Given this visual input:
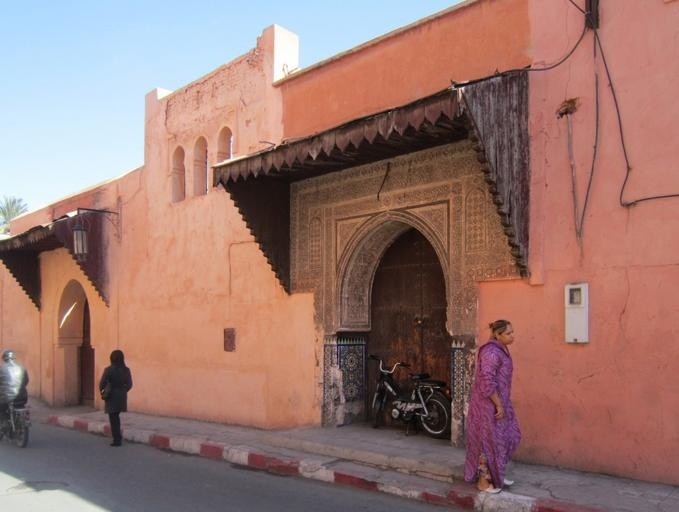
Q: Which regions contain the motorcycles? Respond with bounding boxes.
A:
[0,400,30,447]
[367,354,451,439]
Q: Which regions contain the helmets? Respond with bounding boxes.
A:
[2,350,17,360]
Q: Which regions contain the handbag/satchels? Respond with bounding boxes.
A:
[100,382,114,400]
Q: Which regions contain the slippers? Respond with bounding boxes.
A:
[476,482,502,494]
[501,478,515,486]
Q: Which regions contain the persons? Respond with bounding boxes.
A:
[0,350,29,435]
[99,350,133,447]
[461,319,524,494]
